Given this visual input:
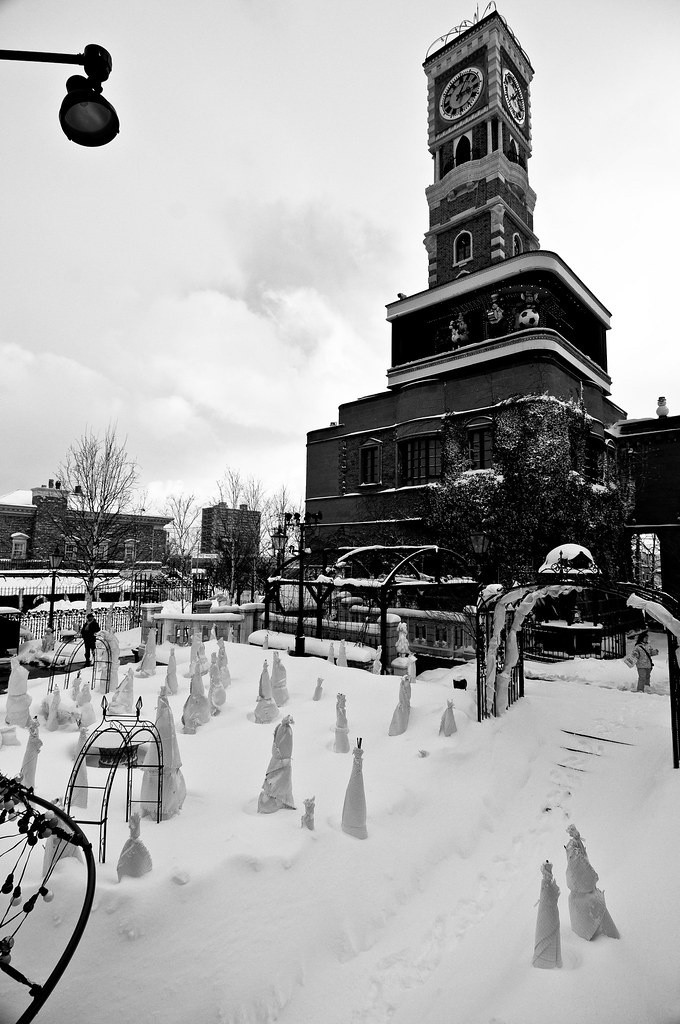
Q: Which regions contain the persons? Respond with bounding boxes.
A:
[81,612,100,662]
[632,632,659,693]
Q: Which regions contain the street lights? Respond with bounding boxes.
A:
[0,43,121,148]
[47,544,65,630]
[270,524,289,615]
